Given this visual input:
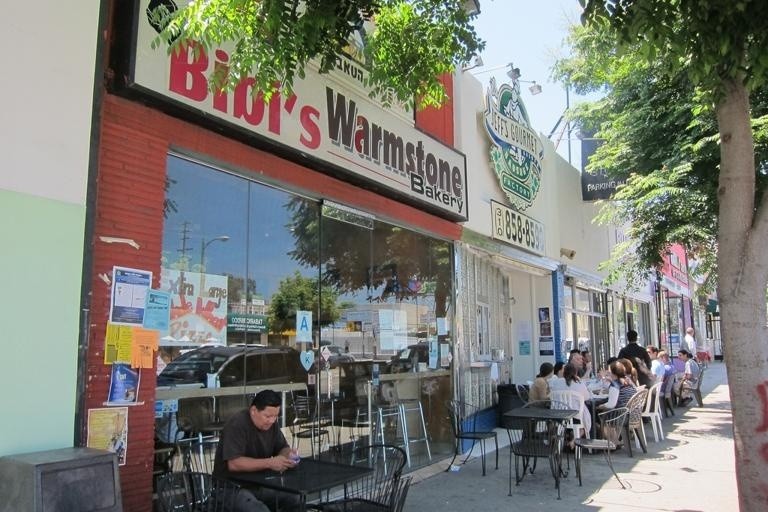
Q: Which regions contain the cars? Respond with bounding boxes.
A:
[309,342,452,425]
[567,336,641,364]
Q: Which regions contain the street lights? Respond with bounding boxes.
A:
[199,235,230,298]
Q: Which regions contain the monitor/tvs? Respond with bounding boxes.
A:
[366,264,397,291]
[398,347,416,361]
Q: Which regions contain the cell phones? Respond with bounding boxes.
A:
[292,458,300,464]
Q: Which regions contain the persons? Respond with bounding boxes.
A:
[408,350,420,374]
[212,390,307,511]
[527,327,700,450]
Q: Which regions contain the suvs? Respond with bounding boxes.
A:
[156,345,318,446]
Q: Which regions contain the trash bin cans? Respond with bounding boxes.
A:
[0,447,123,512]
[496,384,530,429]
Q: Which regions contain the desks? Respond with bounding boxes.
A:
[211,451,380,511]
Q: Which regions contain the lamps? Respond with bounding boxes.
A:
[475,60,523,87]
[522,77,546,97]
[462,54,487,73]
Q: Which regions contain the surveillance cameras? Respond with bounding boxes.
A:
[560,248,577,260]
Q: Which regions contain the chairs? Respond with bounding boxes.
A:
[316,475,413,511]
[443,356,713,503]
[178,435,231,512]
[312,440,415,511]
[152,467,245,511]
[286,372,440,472]
